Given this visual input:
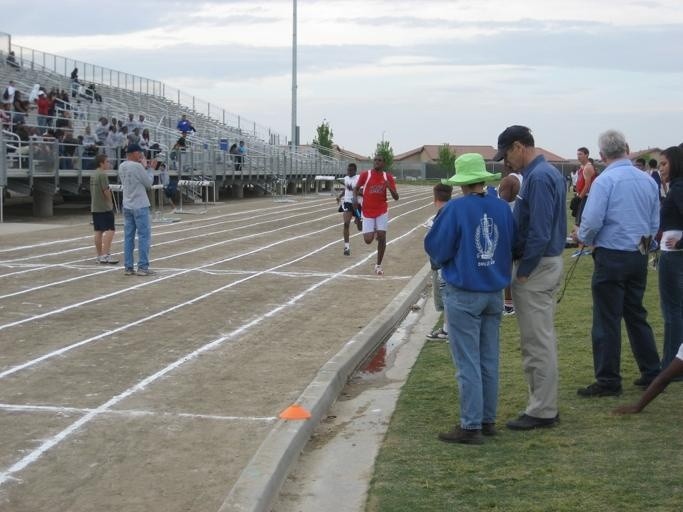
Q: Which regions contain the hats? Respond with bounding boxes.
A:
[492,126,529,162]
[441,152,502,186]
[128,144,145,152]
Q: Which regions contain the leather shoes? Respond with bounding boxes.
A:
[577,383,622,397]
[438,429,481,443]
[633,379,643,386]
[481,423,495,435]
[506,416,557,430]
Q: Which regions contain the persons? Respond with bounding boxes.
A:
[611,341,683,414]
[113,136,161,278]
[635,142,683,383]
[422,149,519,448]
[84,149,120,265]
[565,147,600,258]
[420,181,456,339]
[570,126,664,398]
[333,160,365,255]
[484,168,523,317]
[349,154,398,276]
[0,48,252,202]
[489,123,564,433]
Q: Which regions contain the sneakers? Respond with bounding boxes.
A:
[100,254,119,263]
[137,269,156,276]
[374,264,383,275]
[344,247,349,255]
[96,256,101,264]
[124,266,136,275]
[425,327,446,341]
